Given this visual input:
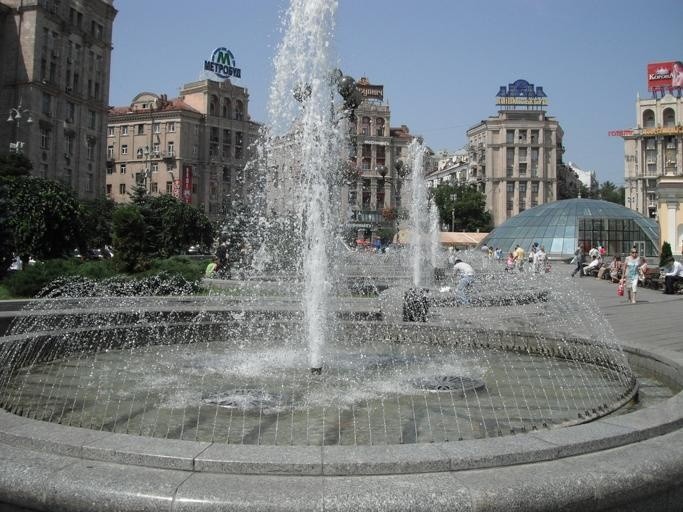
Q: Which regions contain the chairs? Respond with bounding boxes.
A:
[582,262,683,295]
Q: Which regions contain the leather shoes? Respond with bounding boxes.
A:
[663,291,674,294]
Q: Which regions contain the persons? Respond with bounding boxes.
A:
[347,234,395,255]
[451,259,476,305]
[659,256,683,294]
[619,247,647,304]
[446,242,649,288]
[214,237,231,280]
[204,256,219,280]
[670,61,683,88]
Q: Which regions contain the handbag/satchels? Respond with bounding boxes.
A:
[618,285,625,296]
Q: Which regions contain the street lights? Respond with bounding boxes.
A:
[7,95,35,153]
[293,68,363,128]
[377,159,412,247]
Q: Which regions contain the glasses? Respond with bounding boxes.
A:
[632,251,637,254]
